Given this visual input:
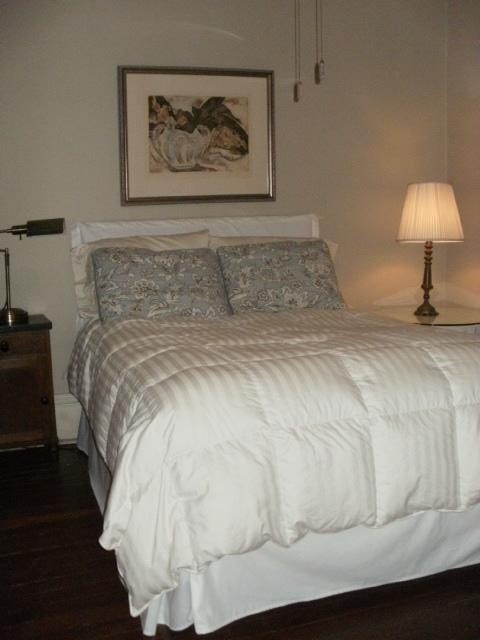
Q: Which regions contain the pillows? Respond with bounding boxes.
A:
[92,247,233,327]
[217,241,347,314]
[69,229,212,319]
[208,235,318,252]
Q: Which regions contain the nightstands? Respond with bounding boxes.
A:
[374,304,479,335]
[0,314,59,453]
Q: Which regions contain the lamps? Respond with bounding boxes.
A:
[0,218,64,326]
[396,181,465,317]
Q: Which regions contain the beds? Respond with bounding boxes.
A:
[71,214,480,636]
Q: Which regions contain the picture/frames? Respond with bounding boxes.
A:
[117,65,276,207]
